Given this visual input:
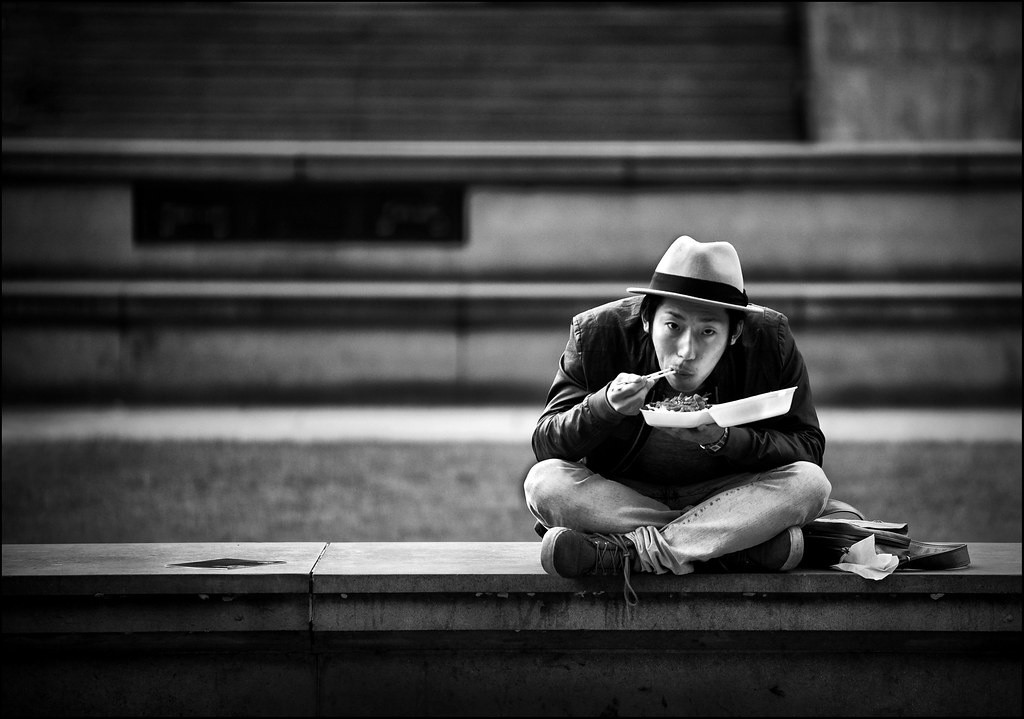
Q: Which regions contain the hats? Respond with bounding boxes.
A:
[625,236,766,315]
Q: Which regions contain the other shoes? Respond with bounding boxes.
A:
[542,526,642,608]
[738,525,805,572]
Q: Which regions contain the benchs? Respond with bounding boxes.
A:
[0,136,1024,272]
[0,544,1023,719]
[2,274,1021,410]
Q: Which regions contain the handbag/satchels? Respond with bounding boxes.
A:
[803,497,972,573]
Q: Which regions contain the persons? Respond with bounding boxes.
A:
[524,235,832,607]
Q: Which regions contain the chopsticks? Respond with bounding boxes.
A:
[611,368,678,390]
